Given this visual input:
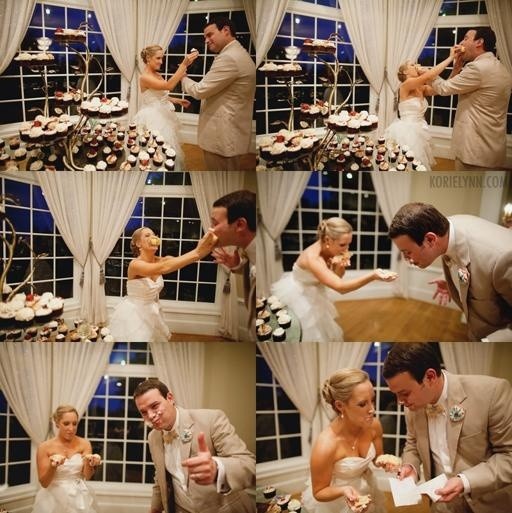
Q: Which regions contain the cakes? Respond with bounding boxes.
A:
[190,48,198,53]
[258,39,426,171]
[255,295,291,342]
[264,486,301,513]
[1,282,114,342]
[149,236,161,247]
[0,27,176,171]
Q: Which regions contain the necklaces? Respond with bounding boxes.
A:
[348,430,364,452]
[62,448,67,452]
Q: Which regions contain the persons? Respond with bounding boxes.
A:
[131,374,256,513]
[301,367,396,513]
[211,189,256,340]
[389,199,512,343]
[382,343,511,512]
[269,214,402,341]
[133,43,200,171]
[386,42,464,171]
[176,14,256,171]
[415,25,512,170]
[107,225,220,341]
[31,403,104,513]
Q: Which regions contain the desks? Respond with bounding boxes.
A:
[1,120,175,171]
[256,294,302,343]
[254,129,424,172]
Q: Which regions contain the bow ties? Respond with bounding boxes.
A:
[424,404,445,419]
[160,429,180,446]
